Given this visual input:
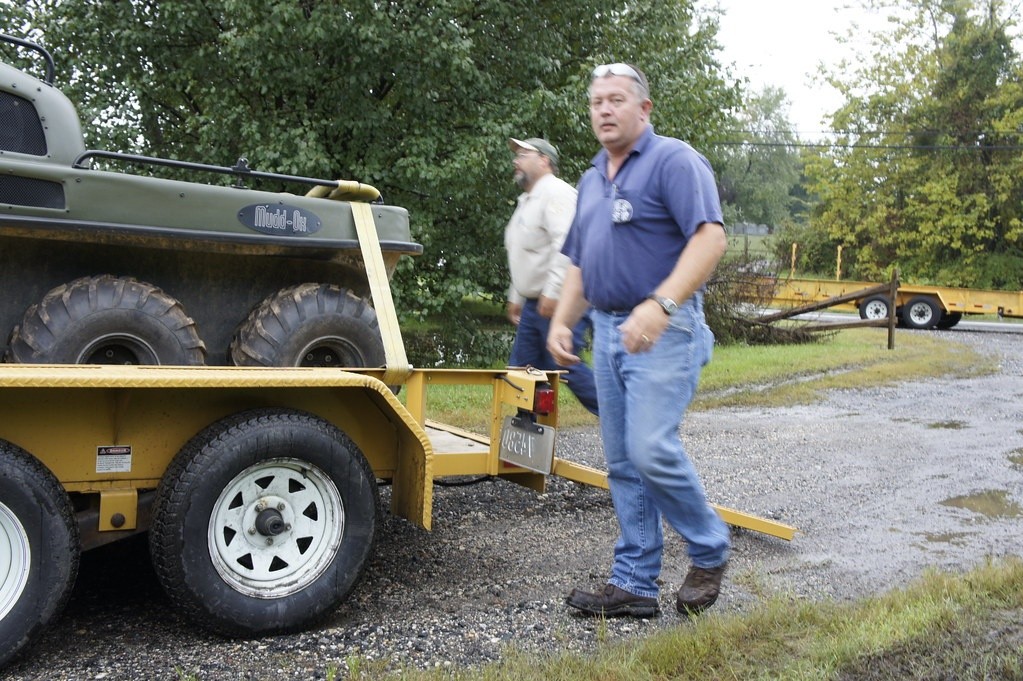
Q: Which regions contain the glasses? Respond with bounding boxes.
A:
[594,63,646,89]
[515,153,544,159]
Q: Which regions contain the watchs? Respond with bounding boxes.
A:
[648,292,678,315]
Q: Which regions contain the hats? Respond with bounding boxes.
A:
[509,137,559,165]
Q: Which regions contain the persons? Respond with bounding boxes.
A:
[502,136,598,417]
[546,63,726,616]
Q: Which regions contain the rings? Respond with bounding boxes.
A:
[643,335,648,342]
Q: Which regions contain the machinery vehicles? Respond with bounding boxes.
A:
[1,32,798,665]
[734,274,1023,328]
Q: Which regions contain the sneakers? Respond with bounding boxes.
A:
[677,562,724,615]
[565,582,661,618]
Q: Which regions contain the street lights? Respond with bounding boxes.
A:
[835,245,843,280]
[790,242,797,278]
